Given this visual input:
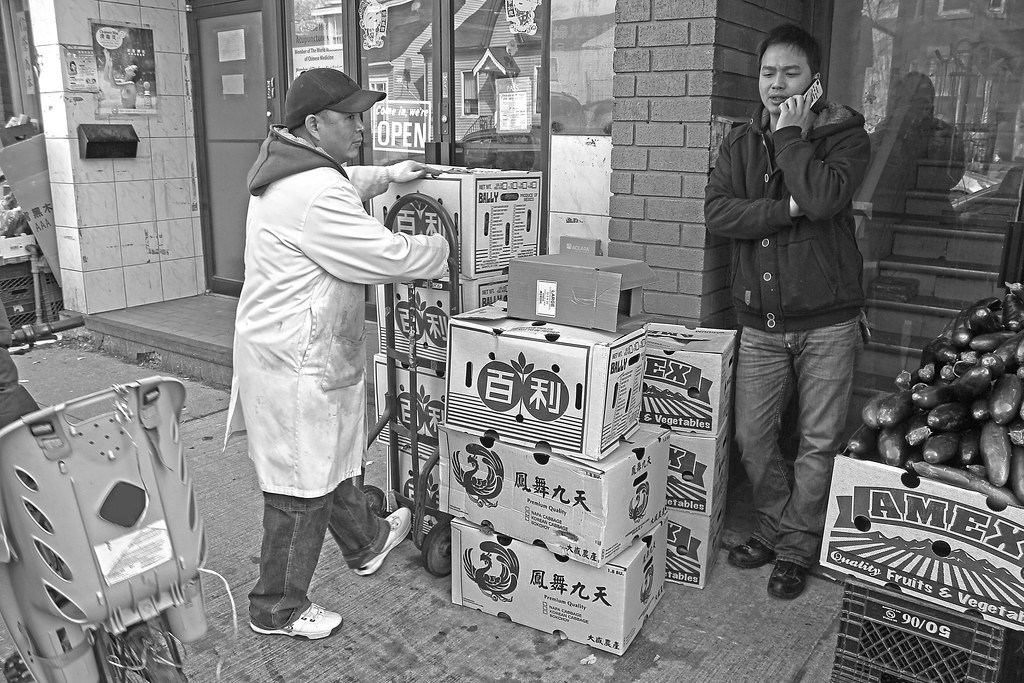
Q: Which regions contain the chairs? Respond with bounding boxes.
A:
[0,374,209,683]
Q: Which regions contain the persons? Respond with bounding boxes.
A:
[0,295,75,682]
[101,49,141,109]
[868,71,966,217]
[233,67,451,642]
[68,61,78,76]
[703,24,872,603]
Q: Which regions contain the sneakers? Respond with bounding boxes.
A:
[353,507,411,576]
[728,536,775,569]
[250,602,343,640]
[767,560,810,600]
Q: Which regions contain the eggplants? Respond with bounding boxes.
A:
[846,278,1024,510]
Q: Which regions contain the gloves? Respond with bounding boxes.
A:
[387,159,440,183]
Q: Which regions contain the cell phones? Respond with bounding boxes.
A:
[795,77,824,110]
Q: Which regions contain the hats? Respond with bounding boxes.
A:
[285,67,387,131]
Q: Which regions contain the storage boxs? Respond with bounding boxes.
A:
[819,449,1024,683]
[0,233,65,332]
[369,170,737,656]
[0,122,36,149]
